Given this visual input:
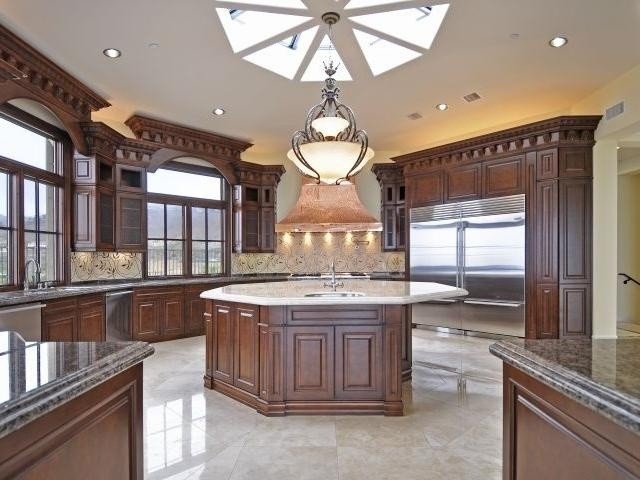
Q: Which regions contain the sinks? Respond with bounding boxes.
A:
[305,291,360,299]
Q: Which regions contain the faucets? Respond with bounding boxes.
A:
[23,258,42,289]
[331,261,336,291]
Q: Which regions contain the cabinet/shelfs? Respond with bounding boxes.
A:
[39,292,107,343]
[242,183,275,251]
[389,111,606,342]
[73,153,146,253]
[186,283,225,338]
[133,288,185,342]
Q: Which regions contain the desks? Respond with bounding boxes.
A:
[488,335,639,479]
[1,329,157,480]
[200,279,469,417]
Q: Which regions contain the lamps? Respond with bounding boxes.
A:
[287,10,375,183]
